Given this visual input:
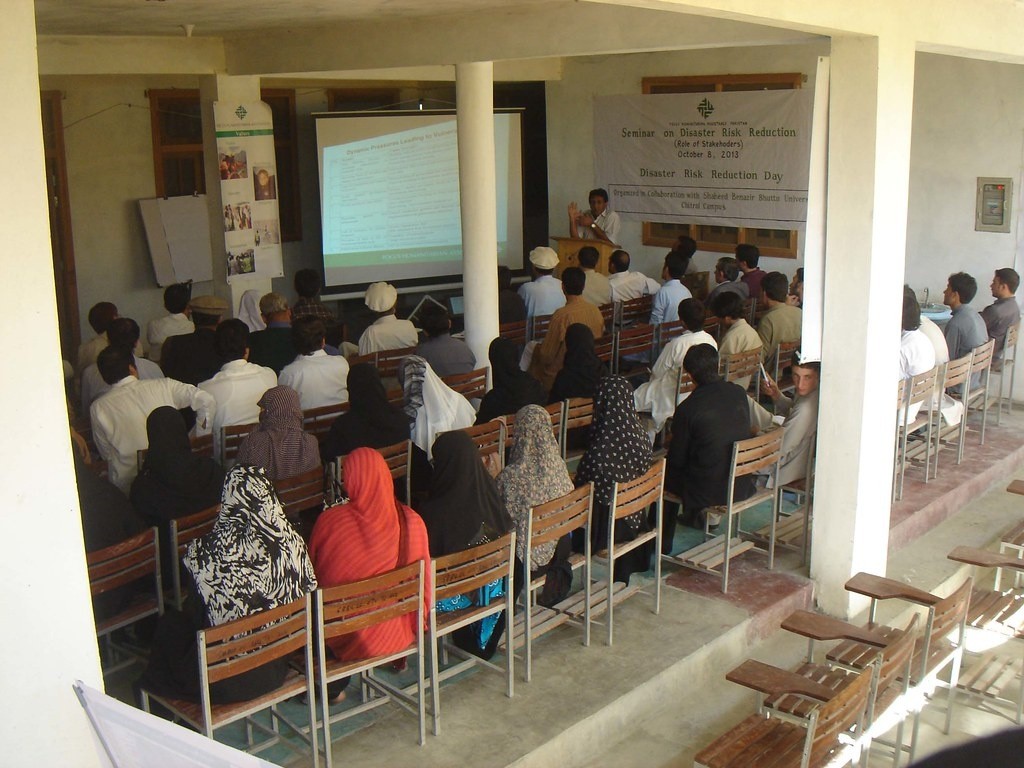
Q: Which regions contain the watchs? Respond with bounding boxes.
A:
[591,224,597,228]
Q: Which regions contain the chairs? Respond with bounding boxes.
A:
[69,271,1024,768]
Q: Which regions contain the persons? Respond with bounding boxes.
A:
[746,347,820,489]
[567,188,621,246]
[496,403,575,607]
[480,237,804,460]
[62,237,476,587]
[574,375,652,587]
[415,430,515,662]
[899,268,1020,442]
[664,343,756,528]
[140,464,317,705]
[308,447,430,703]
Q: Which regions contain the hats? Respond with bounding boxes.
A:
[190,296,228,317]
[529,246,560,269]
[364,282,397,313]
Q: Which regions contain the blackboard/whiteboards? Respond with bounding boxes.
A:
[138,193,214,287]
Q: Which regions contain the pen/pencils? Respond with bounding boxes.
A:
[758,361,770,386]
